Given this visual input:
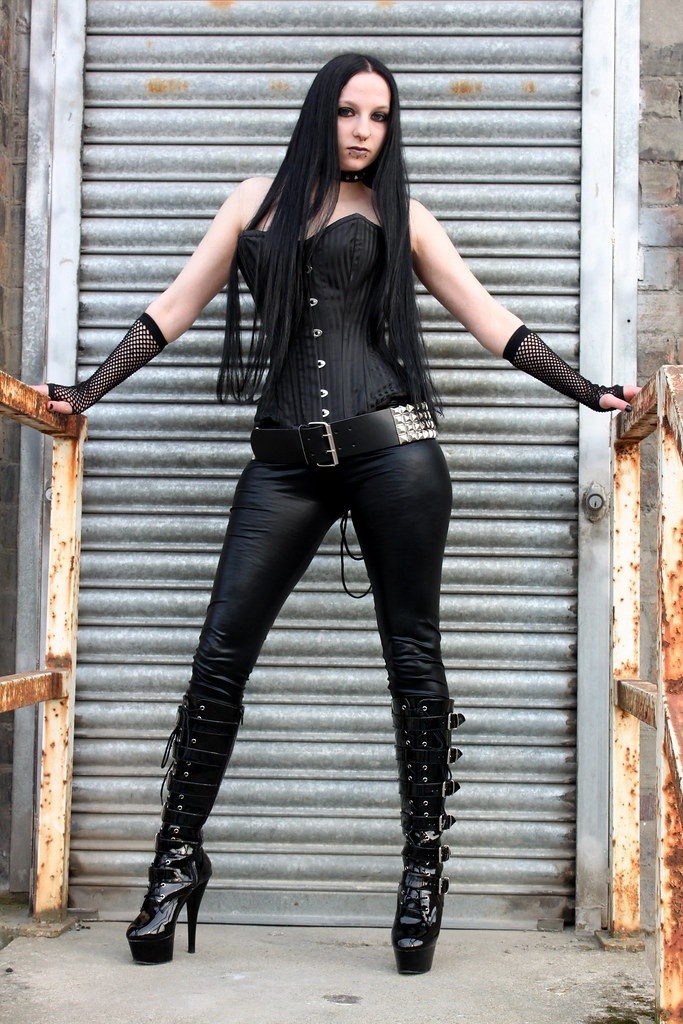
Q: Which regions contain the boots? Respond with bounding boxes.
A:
[390,696,467,974]
[125,697,245,963]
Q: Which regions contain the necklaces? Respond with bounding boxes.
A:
[340,170,368,183]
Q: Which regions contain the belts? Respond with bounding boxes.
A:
[250,401,438,468]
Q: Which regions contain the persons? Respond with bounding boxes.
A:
[24,52,642,974]
